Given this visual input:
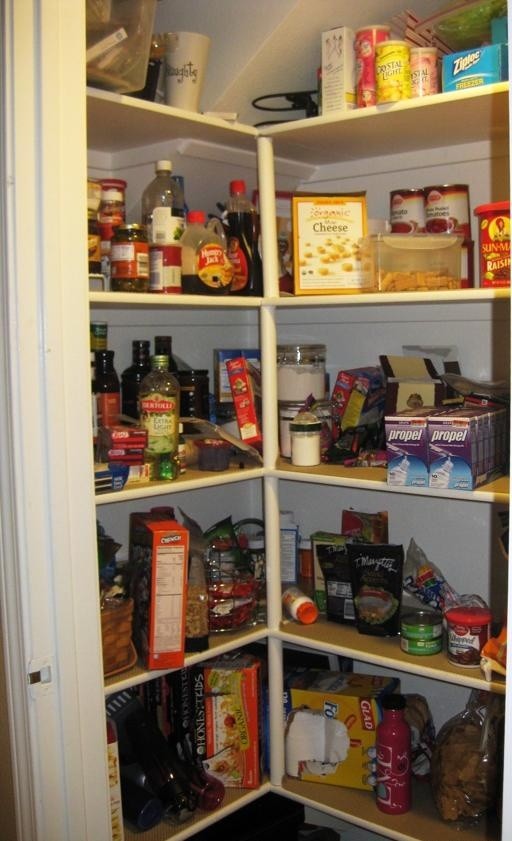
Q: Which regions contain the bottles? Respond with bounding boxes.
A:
[376,695,412,814]
[95,350,120,424]
[219,180,258,293]
[138,355,178,480]
[154,336,177,373]
[90,320,108,392]
[137,159,186,241]
[181,210,231,294]
[282,587,318,623]
[122,340,151,418]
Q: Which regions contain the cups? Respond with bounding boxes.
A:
[165,32,210,112]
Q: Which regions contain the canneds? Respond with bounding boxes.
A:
[402,612,443,654]
[150,243,182,293]
[98,179,126,224]
[88,214,102,273]
[109,223,150,293]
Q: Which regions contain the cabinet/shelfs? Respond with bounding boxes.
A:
[84,67,270,841]
[271,79,509,841]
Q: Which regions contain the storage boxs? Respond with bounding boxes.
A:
[162,660,264,790]
[127,510,190,671]
[283,673,400,792]
